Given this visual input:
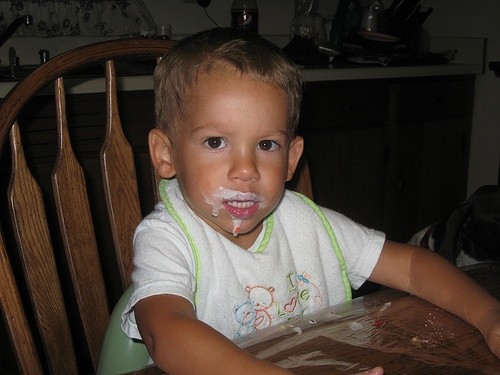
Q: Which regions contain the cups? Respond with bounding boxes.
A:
[154,24,171,38]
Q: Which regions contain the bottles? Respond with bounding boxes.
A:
[231,0,259,36]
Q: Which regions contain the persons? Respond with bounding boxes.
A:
[97,26,500,375]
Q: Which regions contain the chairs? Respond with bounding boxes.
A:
[0,38,314,375]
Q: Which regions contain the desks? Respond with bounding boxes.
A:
[121,262,500,375]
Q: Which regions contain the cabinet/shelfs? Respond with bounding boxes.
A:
[0,64,482,269]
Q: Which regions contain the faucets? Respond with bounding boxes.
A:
[0,14,32,46]
[8,47,17,78]
